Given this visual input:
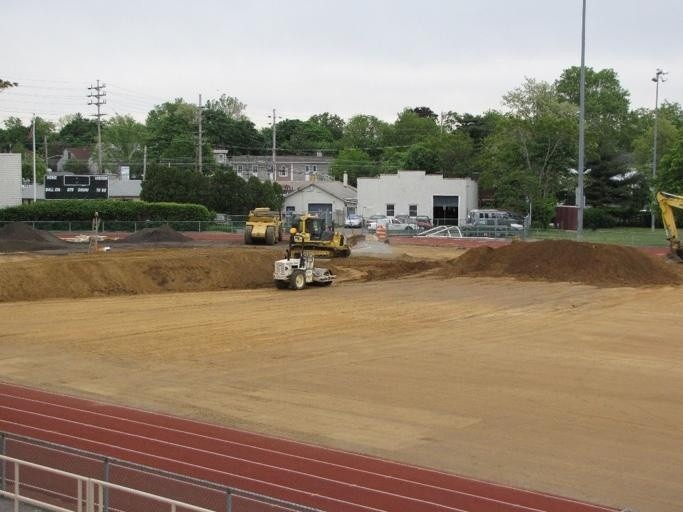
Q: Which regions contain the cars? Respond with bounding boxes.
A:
[364,213,432,235]
[212,212,237,233]
[461,207,523,236]
[343,213,365,229]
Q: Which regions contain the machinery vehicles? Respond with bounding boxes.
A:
[242,205,284,245]
[284,212,352,260]
[271,234,337,291]
[655,188,682,265]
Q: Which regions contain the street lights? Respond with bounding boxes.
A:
[525,195,532,228]
[648,64,671,234]
